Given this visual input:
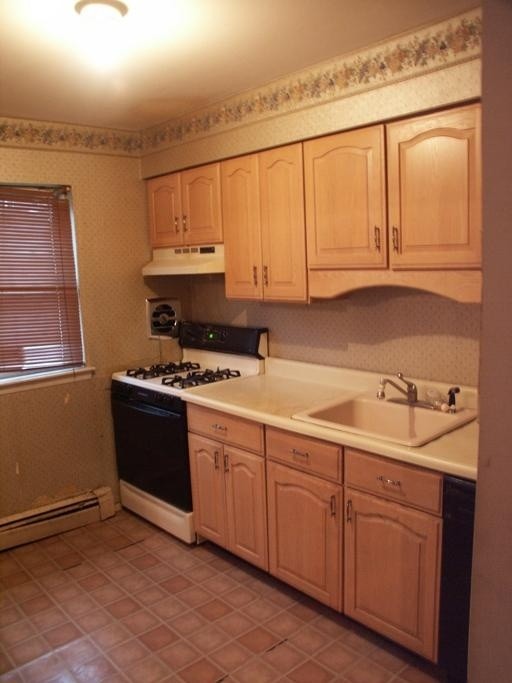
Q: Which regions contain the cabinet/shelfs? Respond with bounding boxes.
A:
[145,161,223,248]
[301,101,480,271]
[263,420,344,616]
[345,442,439,661]
[221,142,310,304]
[186,407,270,571]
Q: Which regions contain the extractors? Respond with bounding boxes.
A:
[143,246,225,276]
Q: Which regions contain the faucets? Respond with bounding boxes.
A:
[376,372,417,403]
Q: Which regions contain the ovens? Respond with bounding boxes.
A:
[110,379,203,545]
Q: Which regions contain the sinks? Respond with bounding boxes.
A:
[289,391,478,447]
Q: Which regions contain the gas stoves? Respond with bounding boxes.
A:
[111,361,242,397]
